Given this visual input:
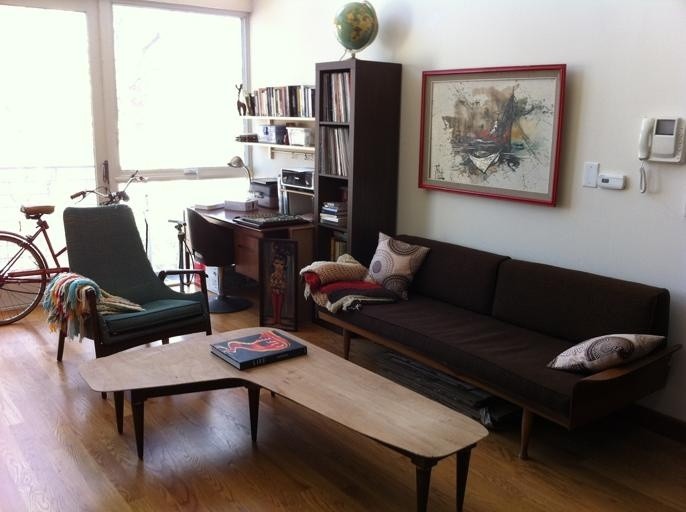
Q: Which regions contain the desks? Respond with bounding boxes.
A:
[195,204,310,325]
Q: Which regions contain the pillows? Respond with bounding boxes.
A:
[363,231,431,302]
[546,333,665,373]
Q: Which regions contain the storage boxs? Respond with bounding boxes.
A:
[250,176,279,209]
[194,256,221,296]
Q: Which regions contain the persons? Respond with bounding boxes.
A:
[268,241,292,325]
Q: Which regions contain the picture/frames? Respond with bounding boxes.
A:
[417,64,566,206]
[257,237,299,330]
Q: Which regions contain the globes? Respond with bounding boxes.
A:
[334,0,377,60]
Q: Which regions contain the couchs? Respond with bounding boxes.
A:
[308,235,671,459]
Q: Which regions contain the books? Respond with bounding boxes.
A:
[320,68,351,264]
[209,327,310,371]
[250,82,316,118]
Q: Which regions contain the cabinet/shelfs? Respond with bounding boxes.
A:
[234,85,315,154]
[317,62,400,266]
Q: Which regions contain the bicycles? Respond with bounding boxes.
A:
[0,170,149,328]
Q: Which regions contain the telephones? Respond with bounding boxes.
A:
[638,116,686,165]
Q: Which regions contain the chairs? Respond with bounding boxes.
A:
[58,206,212,401]
[187,208,251,314]
